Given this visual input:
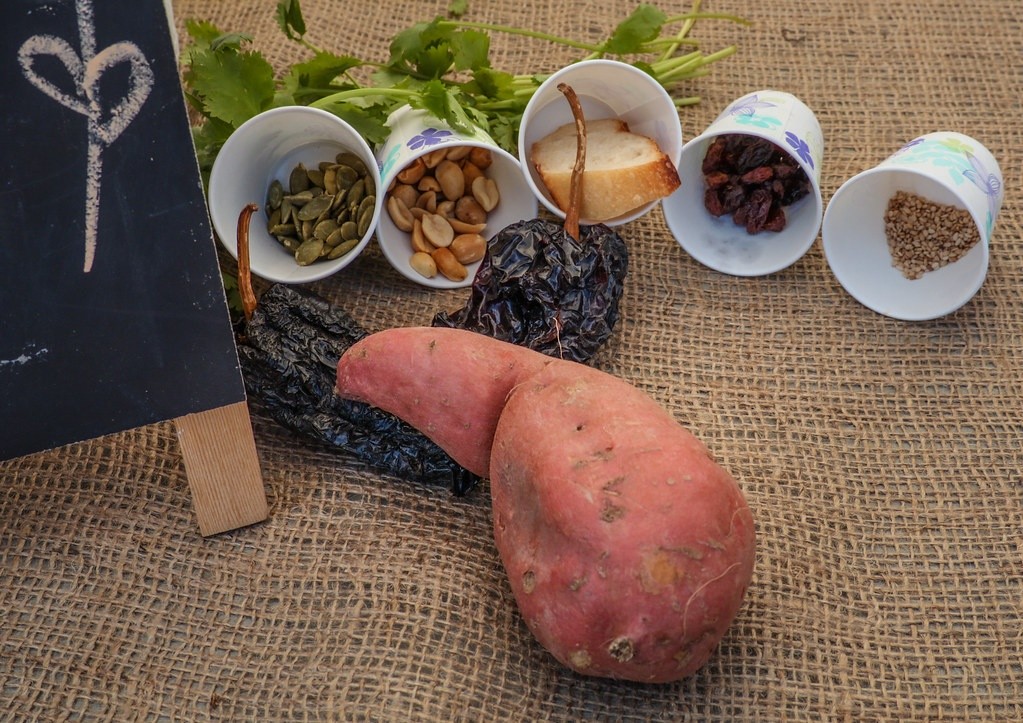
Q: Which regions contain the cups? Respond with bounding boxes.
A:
[374,101,538,290]
[520,60,683,231]
[665,88,827,281]
[207,101,383,286]
[821,129,1006,321]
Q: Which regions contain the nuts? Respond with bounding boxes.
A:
[698,125,799,241]
[262,137,504,288]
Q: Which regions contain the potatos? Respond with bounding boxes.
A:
[333,327,755,680]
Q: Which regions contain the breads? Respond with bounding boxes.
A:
[527,114,680,216]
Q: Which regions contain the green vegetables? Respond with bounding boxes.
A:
[168,0,748,167]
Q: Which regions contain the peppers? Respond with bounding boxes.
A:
[231,81,627,493]
